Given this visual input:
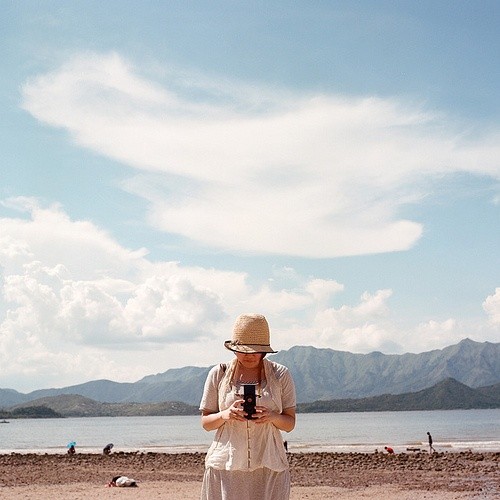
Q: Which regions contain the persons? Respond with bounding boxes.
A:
[198,314,297,500]
[427,432,436,453]
[68,445,75,455]
[385,447,394,454]
[103,443,113,454]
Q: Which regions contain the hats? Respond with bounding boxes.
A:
[224,313,278,354]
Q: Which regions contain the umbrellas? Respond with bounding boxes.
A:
[66,441,76,448]
